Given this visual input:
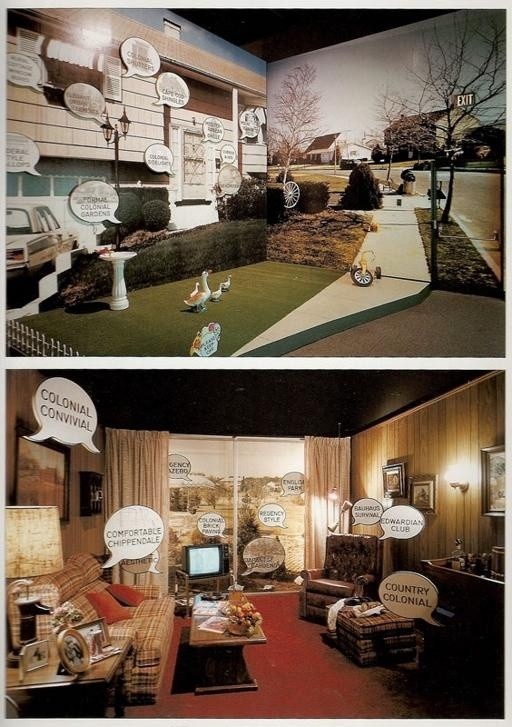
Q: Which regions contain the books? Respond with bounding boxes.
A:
[193,600,231,633]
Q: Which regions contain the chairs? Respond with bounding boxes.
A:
[299,533,383,621]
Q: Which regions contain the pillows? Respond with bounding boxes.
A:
[87,590,132,625]
[107,583,144,606]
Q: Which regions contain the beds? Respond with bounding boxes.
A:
[98,252,137,311]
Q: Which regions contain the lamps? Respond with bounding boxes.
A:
[70,618,111,652]
[449,481,469,492]
[5,505,63,668]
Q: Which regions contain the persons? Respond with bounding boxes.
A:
[64,637,83,659]
[65,649,82,666]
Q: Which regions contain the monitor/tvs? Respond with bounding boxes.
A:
[183,542,230,579]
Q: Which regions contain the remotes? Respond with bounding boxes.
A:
[202,593,225,601]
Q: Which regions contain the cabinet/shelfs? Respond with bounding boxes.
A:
[423,558,504,719]
[78,470,104,517]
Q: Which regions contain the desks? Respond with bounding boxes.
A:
[174,570,233,617]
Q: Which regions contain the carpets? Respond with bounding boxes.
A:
[106,591,422,718]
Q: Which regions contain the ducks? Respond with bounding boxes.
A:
[210,283,223,301]
[190,282,200,298]
[222,274,232,291]
[182,269,212,314]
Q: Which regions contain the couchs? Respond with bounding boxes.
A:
[7,550,174,706]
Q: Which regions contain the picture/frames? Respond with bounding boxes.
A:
[382,463,405,499]
[56,629,89,675]
[408,474,438,516]
[480,444,506,517]
[13,427,70,525]
